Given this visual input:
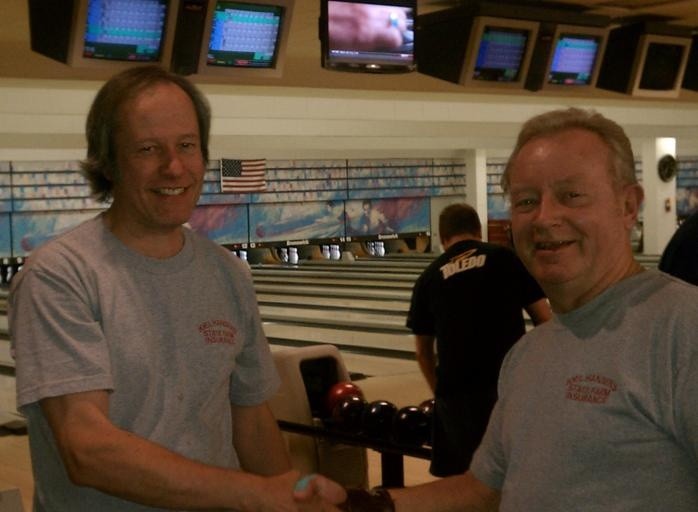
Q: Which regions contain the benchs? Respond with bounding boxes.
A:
[262,342,373,504]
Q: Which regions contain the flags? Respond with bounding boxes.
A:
[219,157,268,192]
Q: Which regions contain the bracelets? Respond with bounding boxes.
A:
[340,483,398,511]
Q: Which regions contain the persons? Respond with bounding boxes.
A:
[403,201,555,485]
[292,106,698,511]
[324,201,351,238]
[7,66,321,511]
[358,200,395,236]
[658,201,698,288]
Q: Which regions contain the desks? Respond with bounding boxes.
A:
[268,402,437,494]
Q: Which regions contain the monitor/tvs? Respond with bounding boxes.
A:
[174,0,295,80]
[524,22,610,93]
[29,0,179,70]
[415,7,540,89]
[595,32,692,99]
[320,0,417,75]
